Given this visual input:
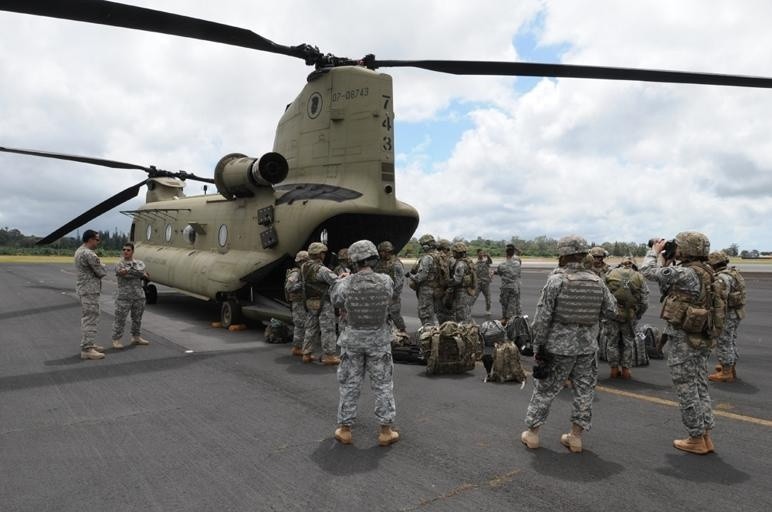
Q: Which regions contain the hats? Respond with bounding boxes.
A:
[418,234,434,246]
[348,240,380,263]
[557,236,589,258]
[337,248,348,259]
[295,251,310,262]
[675,232,710,258]
[622,256,639,270]
[590,247,607,257]
[437,240,451,250]
[377,241,394,253]
[451,242,467,253]
[708,251,729,266]
[308,242,328,255]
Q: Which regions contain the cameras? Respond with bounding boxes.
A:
[648,239,675,259]
[405,268,417,277]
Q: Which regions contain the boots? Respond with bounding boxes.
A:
[378,425,400,446]
[673,434,708,454]
[80,347,104,360]
[704,435,714,451]
[622,367,631,380]
[112,337,125,349]
[291,346,303,358]
[521,425,540,449]
[335,424,352,444]
[715,363,736,377]
[323,353,342,366]
[561,429,582,453]
[130,334,148,345]
[92,343,105,352]
[302,353,320,363]
[610,368,622,379]
[708,365,734,382]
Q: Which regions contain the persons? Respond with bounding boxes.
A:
[520,235,619,452]
[333,248,351,334]
[472,248,493,316]
[643,232,718,455]
[112,242,150,349]
[602,257,651,379]
[300,242,341,364]
[707,251,746,382]
[372,242,406,331]
[285,250,310,355]
[588,247,609,276]
[74,229,107,359]
[408,234,477,327]
[494,245,522,324]
[327,239,400,448]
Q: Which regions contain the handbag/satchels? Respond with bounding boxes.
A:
[417,322,438,364]
[391,336,425,365]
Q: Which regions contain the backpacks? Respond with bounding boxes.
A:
[631,330,651,367]
[598,328,608,360]
[483,339,528,389]
[481,318,506,354]
[416,323,437,361]
[643,324,664,359]
[391,330,419,365]
[503,315,533,354]
[458,320,486,361]
[420,321,476,375]
